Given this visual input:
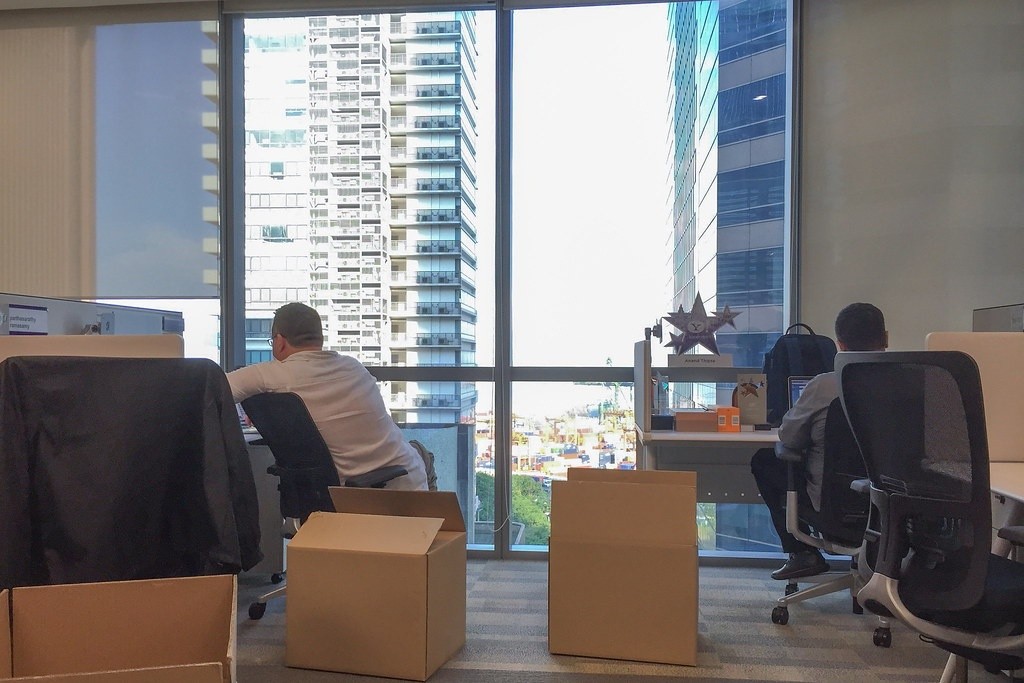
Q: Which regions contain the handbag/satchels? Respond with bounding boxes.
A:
[762,323,838,428]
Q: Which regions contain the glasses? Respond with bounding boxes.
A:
[267,333,287,346]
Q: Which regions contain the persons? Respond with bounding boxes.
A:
[751,302,889,580]
[224,303,429,491]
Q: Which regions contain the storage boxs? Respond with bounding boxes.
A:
[547,468,699,666]
[0,574,238,683]
[286,483,469,681]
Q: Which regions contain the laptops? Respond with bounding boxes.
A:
[788,376,815,411]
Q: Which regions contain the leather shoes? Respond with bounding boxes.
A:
[771,550,830,580]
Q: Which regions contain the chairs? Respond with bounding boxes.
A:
[834,347,1024,683]
[241,392,407,619]
[771,397,920,645]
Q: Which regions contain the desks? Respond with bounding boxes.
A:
[635,423,783,508]
[931,457,1023,565]
